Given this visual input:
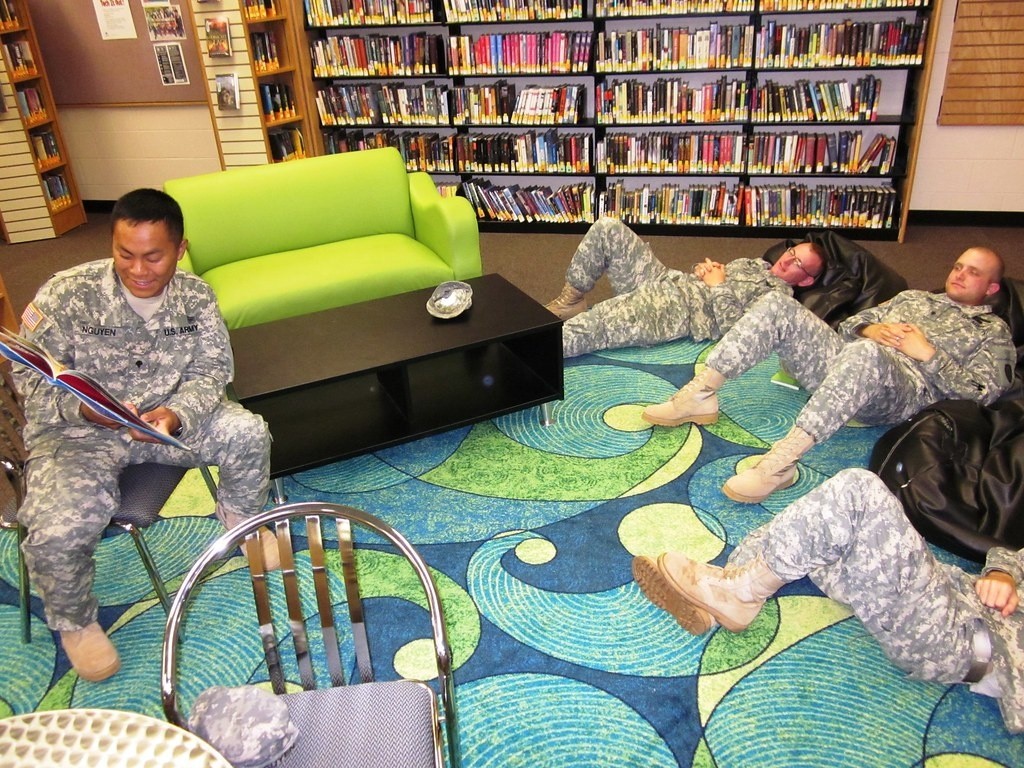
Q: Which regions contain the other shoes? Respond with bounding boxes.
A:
[214,501,281,567]
[60,620,121,681]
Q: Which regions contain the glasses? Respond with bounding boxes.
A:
[786,247,813,278]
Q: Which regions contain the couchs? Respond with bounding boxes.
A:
[162,146,484,331]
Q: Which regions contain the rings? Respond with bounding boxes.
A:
[895,337,900,341]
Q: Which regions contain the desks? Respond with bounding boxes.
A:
[226,273,567,478]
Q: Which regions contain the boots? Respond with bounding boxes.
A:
[642,366,725,426]
[722,426,816,502]
[633,555,717,635]
[659,552,786,632]
[545,281,586,321]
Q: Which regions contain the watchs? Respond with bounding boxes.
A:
[174,424,183,435]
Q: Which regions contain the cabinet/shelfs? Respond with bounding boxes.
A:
[189,0,942,243]
[0,0,87,244]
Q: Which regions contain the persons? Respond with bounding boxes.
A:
[0,190,272,679]
[631,467,1023,737]
[147,5,183,37]
[546,216,829,358]
[640,245,1018,499]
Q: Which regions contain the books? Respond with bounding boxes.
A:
[1,330,194,454]
[304,0,933,232]
[215,73,240,111]
[771,367,801,391]
[0,0,73,211]
[204,15,232,58]
[243,0,304,162]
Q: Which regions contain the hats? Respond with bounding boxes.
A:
[187,685,301,768]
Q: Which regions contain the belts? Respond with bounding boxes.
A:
[963,618,991,683]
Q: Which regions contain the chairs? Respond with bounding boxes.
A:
[162,502,463,768]
[0,359,219,642]
